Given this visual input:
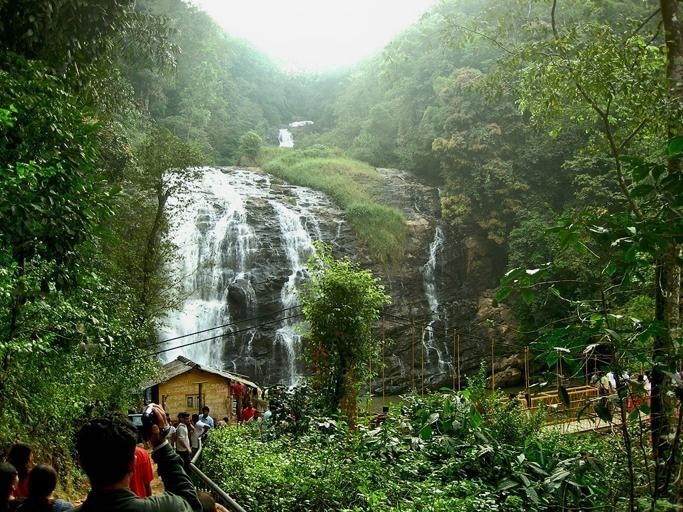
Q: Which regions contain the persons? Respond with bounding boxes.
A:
[376,406,390,426]
[504,392,533,421]
[0,403,229,512]
[242,386,286,424]
[589,367,683,415]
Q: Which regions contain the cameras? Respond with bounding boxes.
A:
[127,403,156,431]
